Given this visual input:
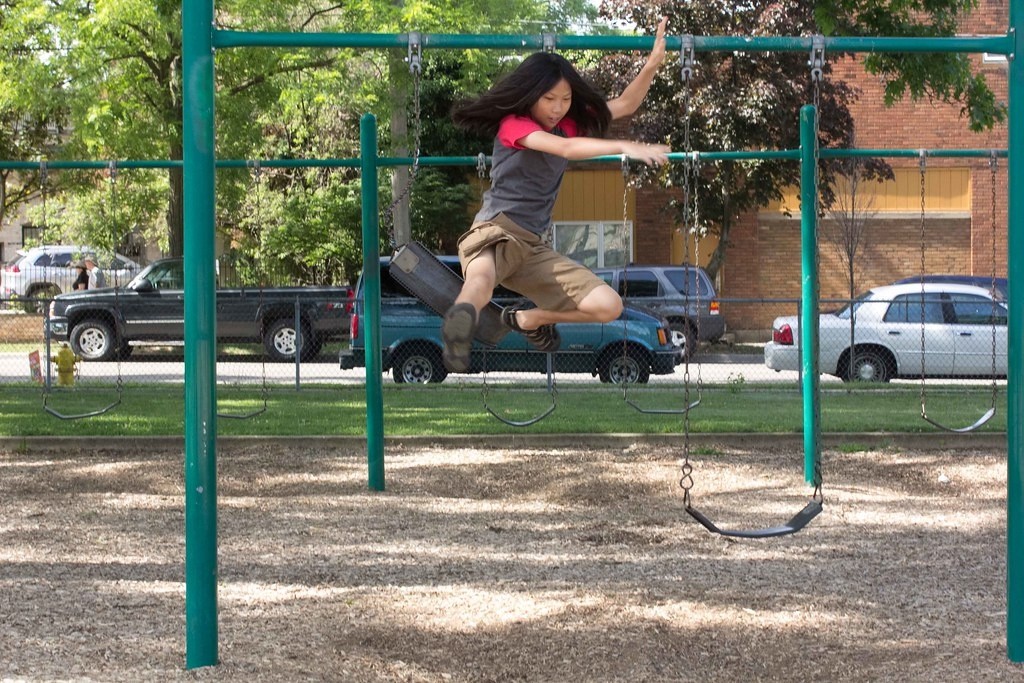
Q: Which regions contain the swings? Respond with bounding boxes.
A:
[677,51,832,540]
[619,163,704,416]
[475,176,559,430]
[385,48,516,348]
[917,155,1000,436]
[38,172,121,422]
[214,171,270,422]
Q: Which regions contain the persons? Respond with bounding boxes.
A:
[441,14,671,374]
[73,259,88,292]
[84,255,106,290]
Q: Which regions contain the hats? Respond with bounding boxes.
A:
[75,260,87,269]
[85,254,98,266]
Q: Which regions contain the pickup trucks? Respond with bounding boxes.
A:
[44,257,353,363]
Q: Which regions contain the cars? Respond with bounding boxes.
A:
[853,276,1009,325]
[764,283,1008,383]
[0,245,170,313]
[339,255,682,384]
[590,262,724,360]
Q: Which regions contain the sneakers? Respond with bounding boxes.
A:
[440,303,477,373]
[500,306,562,353]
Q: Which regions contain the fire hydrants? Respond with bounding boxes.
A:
[49,343,81,387]
